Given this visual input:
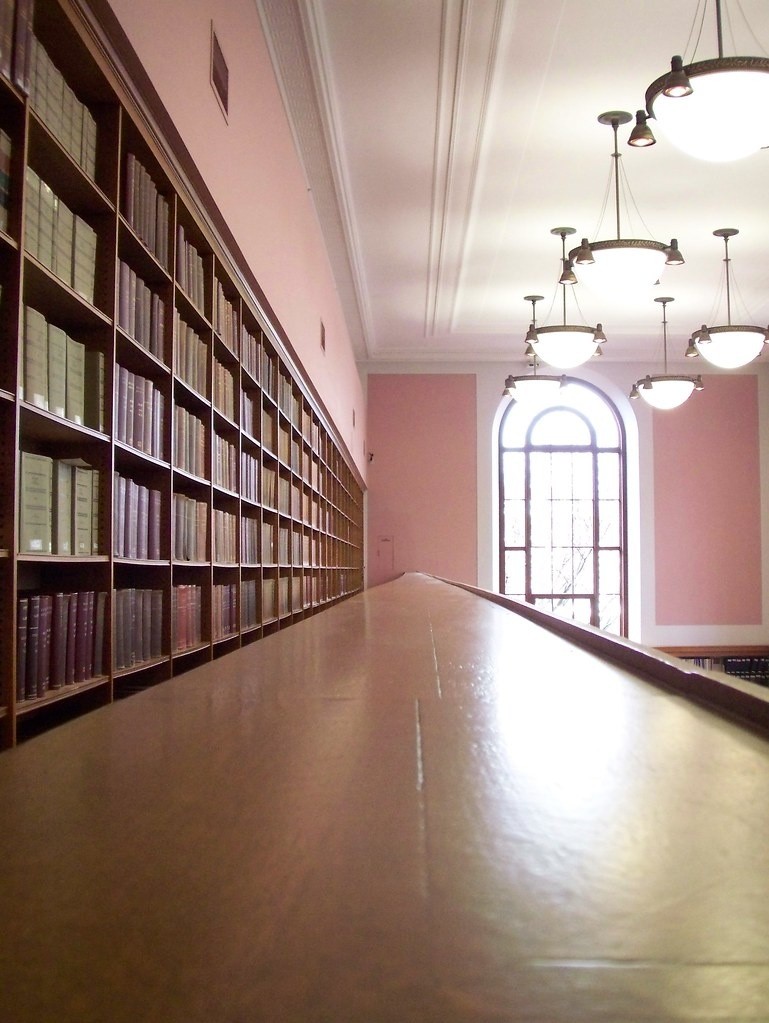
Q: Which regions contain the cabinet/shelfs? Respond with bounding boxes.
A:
[0,0,368,739]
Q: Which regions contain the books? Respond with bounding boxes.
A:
[683,657,769,685]
[0,1,346,707]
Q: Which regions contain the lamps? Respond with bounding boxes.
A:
[628,0,769,165]
[559,111,685,298]
[627,295,703,412]
[501,358,564,412]
[684,229,769,376]
[522,228,606,375]
